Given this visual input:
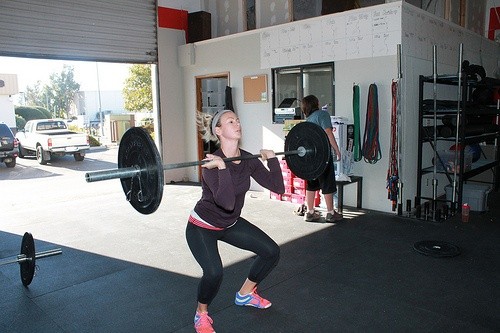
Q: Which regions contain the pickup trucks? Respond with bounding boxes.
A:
[15,119,91,165]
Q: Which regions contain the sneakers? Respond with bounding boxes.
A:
[325,209,343,221]
[234,286,272,309]
[305,212,321,222]
[193,311,216,333]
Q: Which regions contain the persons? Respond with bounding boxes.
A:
[185,109,286,333]
[301,94,344,224]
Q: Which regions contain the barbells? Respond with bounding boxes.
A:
[85,120,332,216]
[0,232,63,288]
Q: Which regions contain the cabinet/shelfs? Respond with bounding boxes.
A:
[416,70,500,219]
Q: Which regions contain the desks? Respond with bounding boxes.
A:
[335,176,362,214]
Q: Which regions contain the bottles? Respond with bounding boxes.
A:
[462,203,470,223]
[322,106,327,111]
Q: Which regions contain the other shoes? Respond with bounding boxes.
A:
[294,204,318,216]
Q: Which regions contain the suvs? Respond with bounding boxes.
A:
[0,123,19,168]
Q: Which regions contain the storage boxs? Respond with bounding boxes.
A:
[270,120,321,208]
[434,145,496,212]
